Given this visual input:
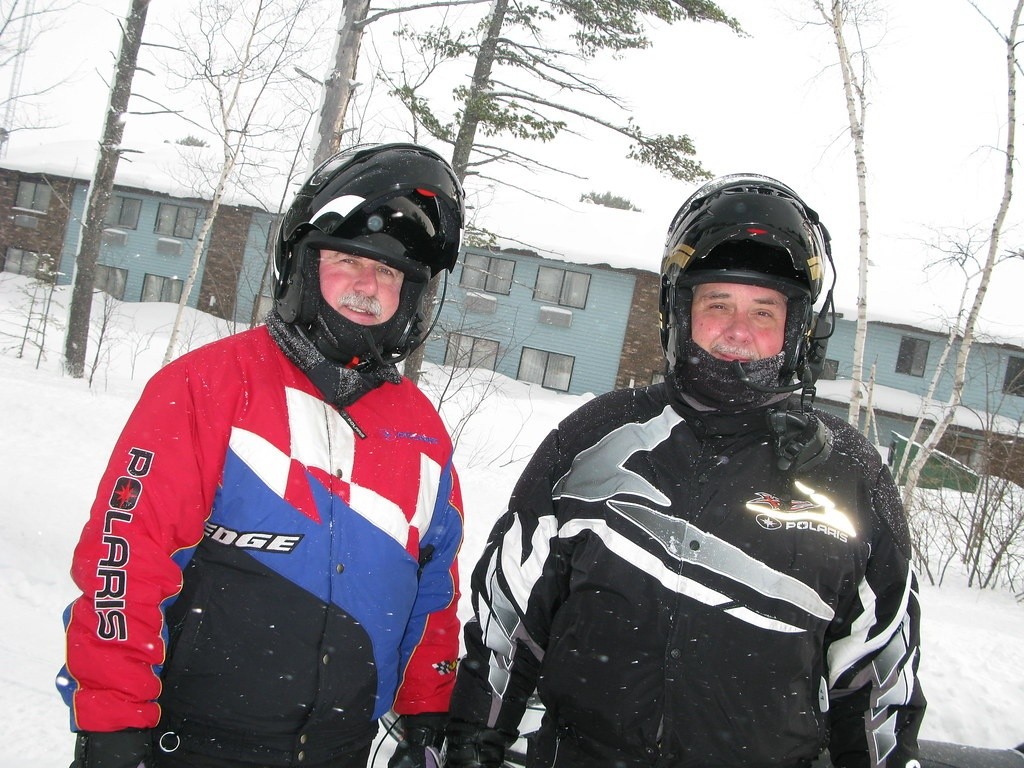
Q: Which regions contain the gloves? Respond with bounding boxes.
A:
[448,720,520,768]
[387,715,449,768]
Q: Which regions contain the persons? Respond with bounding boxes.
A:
[56,143,465,768]
[390,175,925,768]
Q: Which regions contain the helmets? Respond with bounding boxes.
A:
[659,173,824,374]
[271,141,466,354]
